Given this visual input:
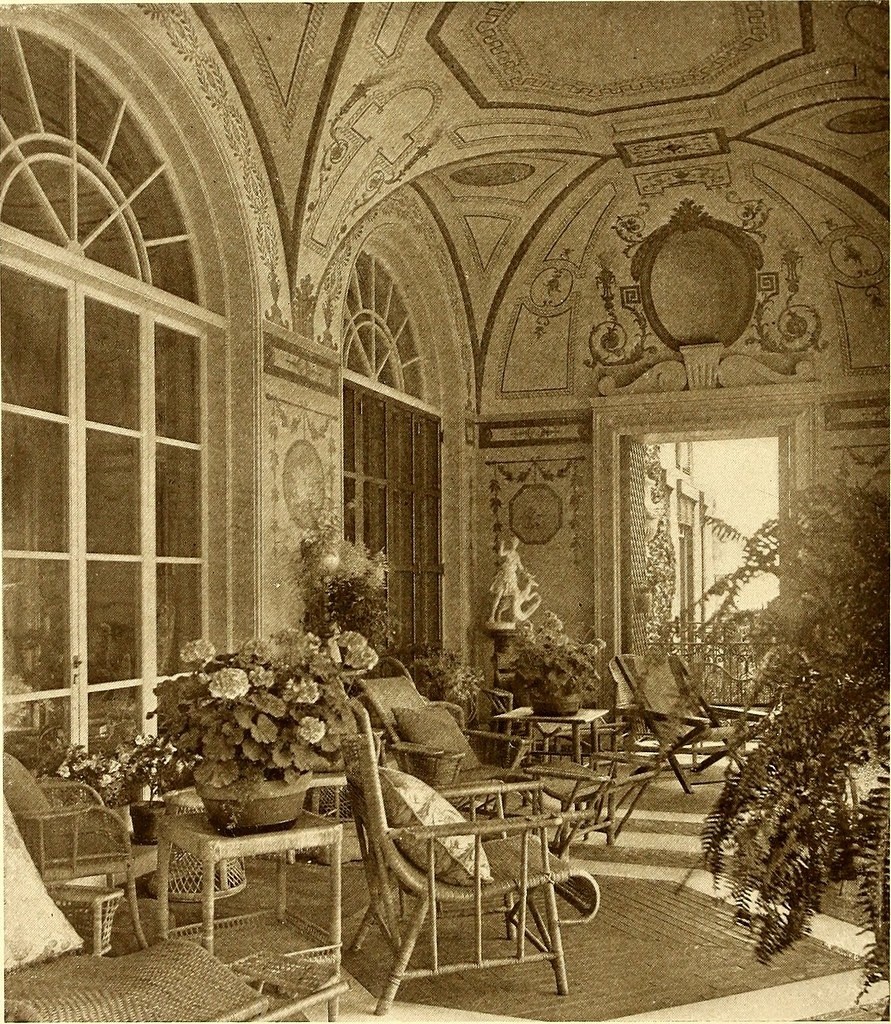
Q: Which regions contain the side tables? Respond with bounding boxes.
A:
[489,703,625,866]
[154,800,349,1002]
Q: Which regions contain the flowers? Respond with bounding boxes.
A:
[495,608,608,695]
[141,624,379,790]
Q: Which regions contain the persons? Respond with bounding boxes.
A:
[484,534,530,627]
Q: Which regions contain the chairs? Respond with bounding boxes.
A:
[343,656,572,864]
[608,648,770,796]
[337,698,602,1018]
[3,751,151,957]
[2,794,273,1024]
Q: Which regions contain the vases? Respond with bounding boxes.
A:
[527,688,582,715]
[192,767,315,839]
